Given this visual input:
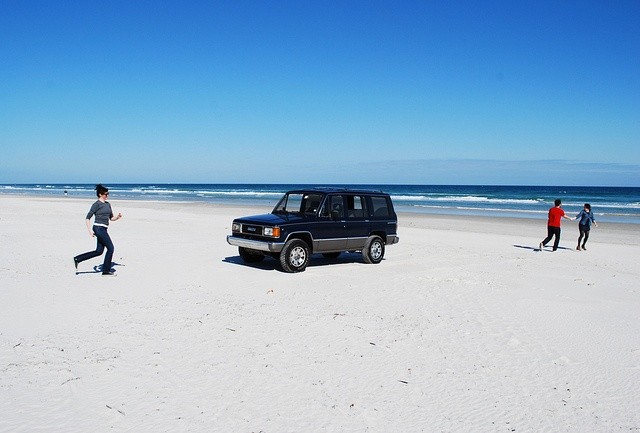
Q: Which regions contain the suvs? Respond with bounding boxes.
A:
[226,188,399,271]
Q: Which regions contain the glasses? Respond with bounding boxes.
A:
[584,207,587,208]
[101,193,109,197]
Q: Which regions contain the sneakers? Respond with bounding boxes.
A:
[540,243,543,251]
[74,257,78,269]
[553,248,557,251]
[577,246,581,250]
[582,245,586,250]
[102,271,117,276]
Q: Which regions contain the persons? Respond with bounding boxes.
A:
[540,199,572,253]
[74,185,122,276]
[572,203,598,251]
